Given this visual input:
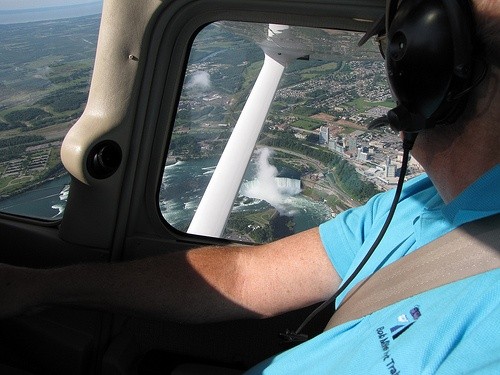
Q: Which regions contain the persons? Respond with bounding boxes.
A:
[0,0,500,375]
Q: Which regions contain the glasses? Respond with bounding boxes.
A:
[374,33,387,60]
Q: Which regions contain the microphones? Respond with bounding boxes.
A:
[368,106,409,131]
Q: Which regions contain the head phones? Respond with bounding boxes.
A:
[387,0,480,134]
